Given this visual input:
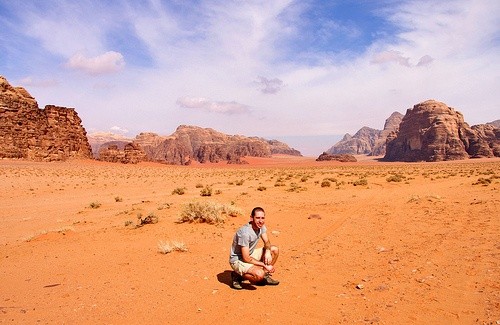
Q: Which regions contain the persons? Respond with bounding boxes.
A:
[229,207,279,290]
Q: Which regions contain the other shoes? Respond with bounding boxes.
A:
[264,277,280,285]
[231,271,242,289]
